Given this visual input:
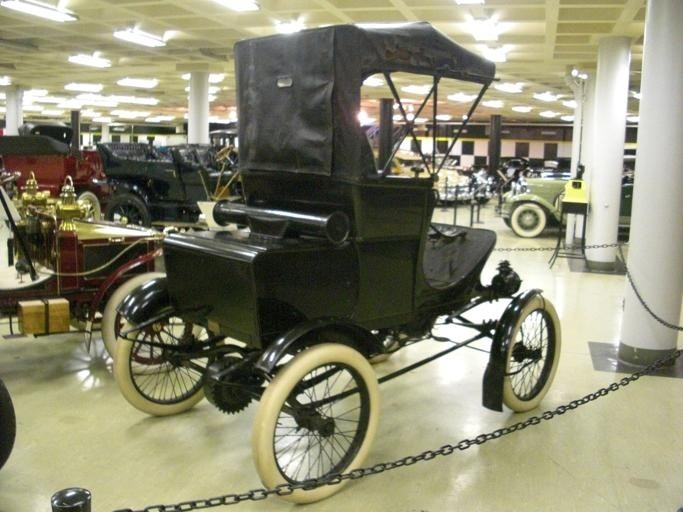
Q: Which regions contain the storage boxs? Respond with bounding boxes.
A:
[18,298,70,335]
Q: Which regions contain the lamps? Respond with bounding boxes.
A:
[1,0,645,127]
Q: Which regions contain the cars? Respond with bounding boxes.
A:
[0,121,242,367]
[371,143,633,238]
